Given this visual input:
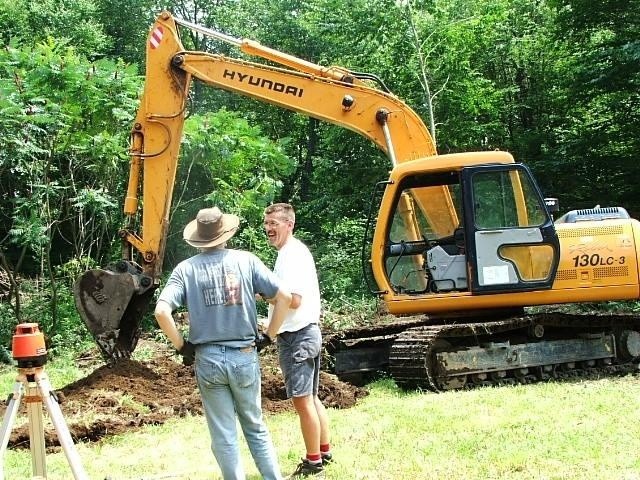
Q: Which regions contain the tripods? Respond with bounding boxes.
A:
[0,367,87,480]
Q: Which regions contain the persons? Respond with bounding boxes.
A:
[154,207,294,480]
[255,203,335,477]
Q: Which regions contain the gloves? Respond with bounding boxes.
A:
[255,333,272,353]
[175,339,196,366]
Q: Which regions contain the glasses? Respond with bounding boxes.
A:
[263,220,289,229]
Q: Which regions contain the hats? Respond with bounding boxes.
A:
[183,206,239,249]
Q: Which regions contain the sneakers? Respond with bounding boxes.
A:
[288,454,334,480]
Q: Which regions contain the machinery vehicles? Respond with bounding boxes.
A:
[70,12,639,388]
